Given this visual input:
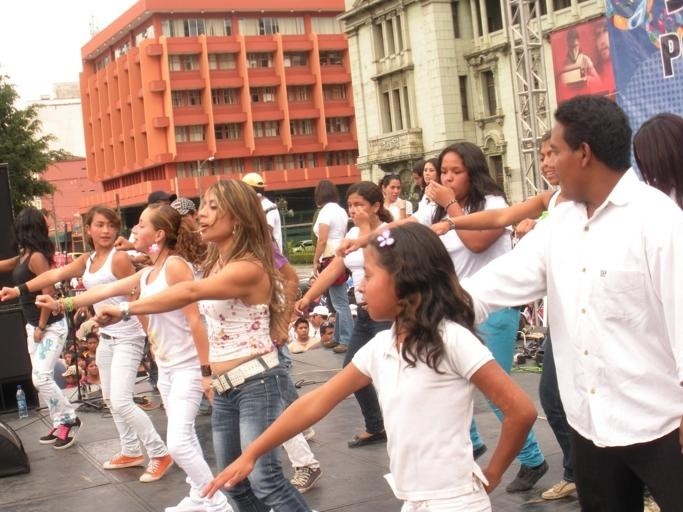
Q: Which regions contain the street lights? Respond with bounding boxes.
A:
[196,155,214,205]
[72,211,86,253]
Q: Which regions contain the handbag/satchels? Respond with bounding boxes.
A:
[320,255,352,287]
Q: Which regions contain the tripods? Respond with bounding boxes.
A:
[35,252,104,411]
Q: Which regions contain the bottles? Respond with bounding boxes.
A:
[15,385,28,418]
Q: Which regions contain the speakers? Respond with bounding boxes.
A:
[0,418,31,479]
[0,302,33,391]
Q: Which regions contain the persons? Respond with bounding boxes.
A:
[557,30,601,89]
[0,94,683,512]
[589,24,613,76]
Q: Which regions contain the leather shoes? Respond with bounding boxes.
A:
[347,429,389,448]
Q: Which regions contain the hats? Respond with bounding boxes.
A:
[309,303,359,316]
[241,171,266,188]
[148,190,197,217]
[61,365,83,377]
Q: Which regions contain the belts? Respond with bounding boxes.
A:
[209,351,279,397]
[99,332,116,341]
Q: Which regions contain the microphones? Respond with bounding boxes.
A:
[63,222,69,252]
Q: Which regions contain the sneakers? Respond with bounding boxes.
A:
[504,456,548,494]
[39,426,58,444]
[54,415,83,450]
[103,453,145,470]
[140,452,174,482]
[333,344,349,353]
[163,495,207,512]
[324,339,338,348]
[290,466,324,493]
[541,479,584,501]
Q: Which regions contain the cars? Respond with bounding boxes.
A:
[289,240,314,255]
